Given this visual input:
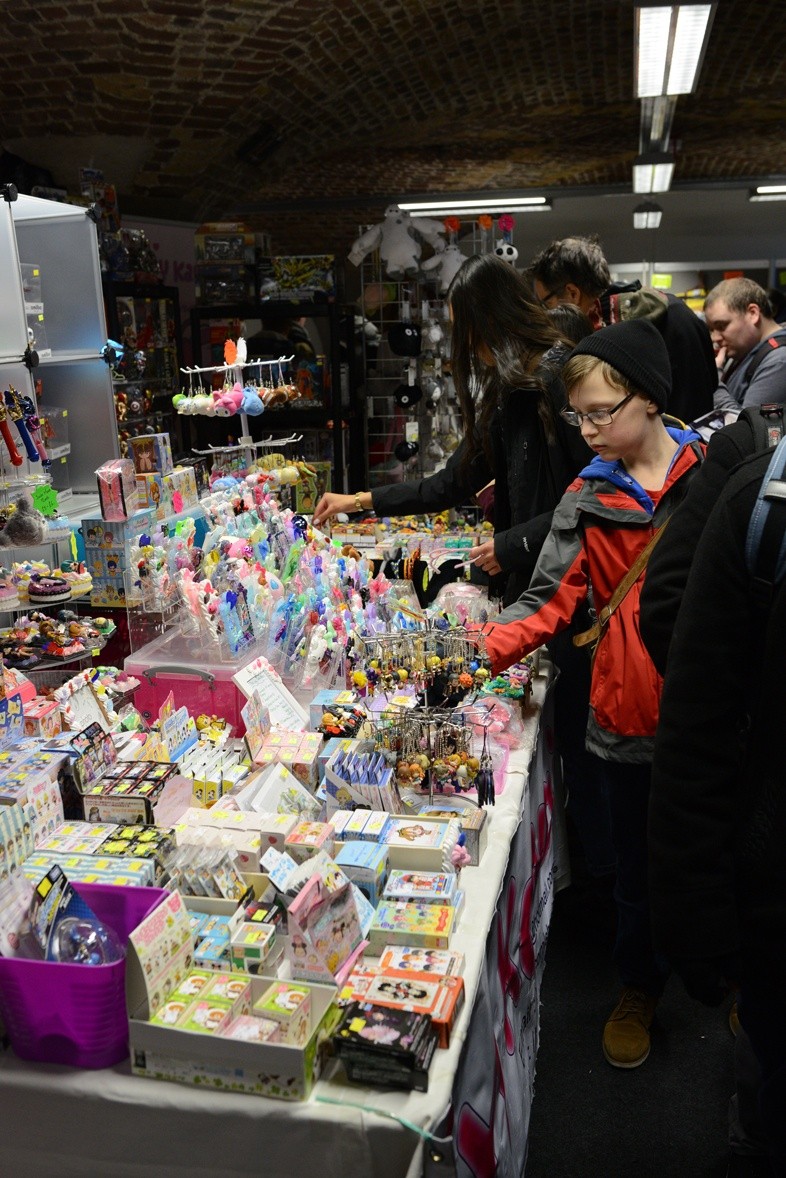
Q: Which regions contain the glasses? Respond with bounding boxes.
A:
[560,386,639,427]
[539,286,562,310]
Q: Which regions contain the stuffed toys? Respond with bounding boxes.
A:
[349,208,525,294]
[171,377,300,417]
[0,493,48,548]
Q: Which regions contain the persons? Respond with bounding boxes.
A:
[522,237,720,437]
[637,389,786,699]
[312,252,626,941]
[240,313,325,426]
[411,319,753,1071]
[326,306,379,484]
[695,276,786,439]
[649,430,786,1178]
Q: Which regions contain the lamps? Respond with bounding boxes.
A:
[633,0,721,231]
[395,197,554,216]
[748,184,786,204]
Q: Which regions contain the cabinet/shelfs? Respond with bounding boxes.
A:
[191,300,368,492]
[353,220,484,484]
[1,477,113,675]
[180,354,304,468]
[112,376,179,424]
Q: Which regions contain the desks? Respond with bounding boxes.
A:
[0,652,565,1178]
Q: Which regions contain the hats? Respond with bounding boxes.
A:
[569,319,672,415]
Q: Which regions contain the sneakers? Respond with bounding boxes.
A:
[603,988,657,1068]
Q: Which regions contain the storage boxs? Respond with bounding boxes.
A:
[20,261,76,501]
[124,618,255,738]
[1,880,166,1069]
[121,888,338,1102]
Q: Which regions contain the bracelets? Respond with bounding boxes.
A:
[353,490,366,512]
